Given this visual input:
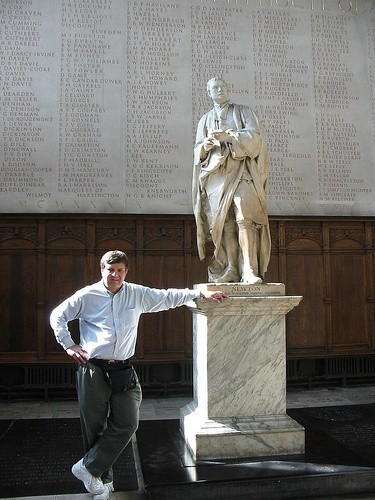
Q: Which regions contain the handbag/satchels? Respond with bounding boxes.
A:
[88,361,137,392]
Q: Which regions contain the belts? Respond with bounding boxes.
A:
[96,360,129,365]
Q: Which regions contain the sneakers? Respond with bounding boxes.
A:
[93,480,115,500]
[71,458,105,494]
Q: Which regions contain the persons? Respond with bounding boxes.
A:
[49,249,229,500]
[192,76,273,286]
